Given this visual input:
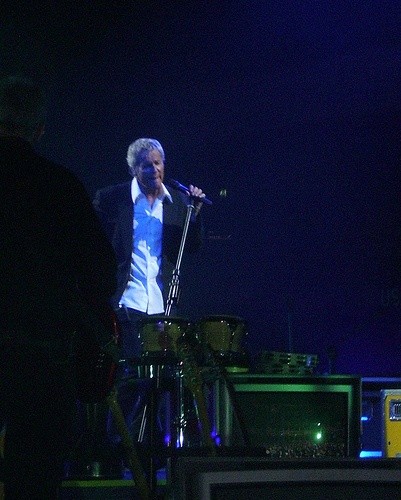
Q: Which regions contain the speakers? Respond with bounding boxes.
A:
[212,372,361,460]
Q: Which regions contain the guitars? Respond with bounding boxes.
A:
[72,295,122,404]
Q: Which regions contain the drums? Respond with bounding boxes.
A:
[191,314,246,369]
[136,315,187,367]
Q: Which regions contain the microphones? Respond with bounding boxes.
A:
[167,179,213,206]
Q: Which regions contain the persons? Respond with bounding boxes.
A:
[91,135,212,473]
[1,73,117,500]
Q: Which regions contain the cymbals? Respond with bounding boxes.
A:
[261,351,321,364]
[263,367,314,376]
